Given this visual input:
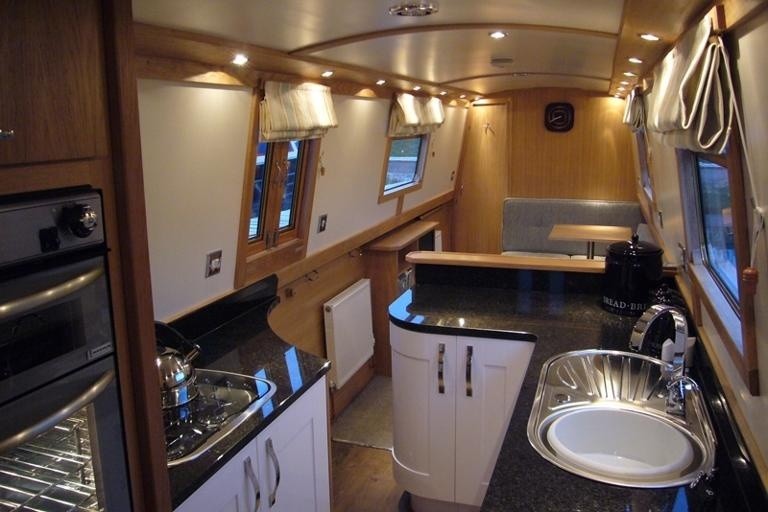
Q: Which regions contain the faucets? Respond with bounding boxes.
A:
[628,303,687,418]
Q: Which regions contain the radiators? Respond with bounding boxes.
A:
[324,276,375,391]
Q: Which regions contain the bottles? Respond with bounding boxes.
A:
[601,232,664,317]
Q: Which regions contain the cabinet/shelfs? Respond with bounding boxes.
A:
[170,375,331,512]
[1,0,137,194]
[360,221,442,378]
[390,320,537,508]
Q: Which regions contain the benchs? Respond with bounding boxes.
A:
[500,198,657,265]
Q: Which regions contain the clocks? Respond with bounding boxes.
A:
[545,102,574,133]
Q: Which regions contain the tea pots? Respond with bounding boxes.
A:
[155,341,202,409]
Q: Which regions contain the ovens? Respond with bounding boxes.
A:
[0,187,131,512]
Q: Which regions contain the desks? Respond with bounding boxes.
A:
[547,221,634,260]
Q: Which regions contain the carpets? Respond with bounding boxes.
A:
[332,376,394,454]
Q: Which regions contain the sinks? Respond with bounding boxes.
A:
[537,403,707,484]
